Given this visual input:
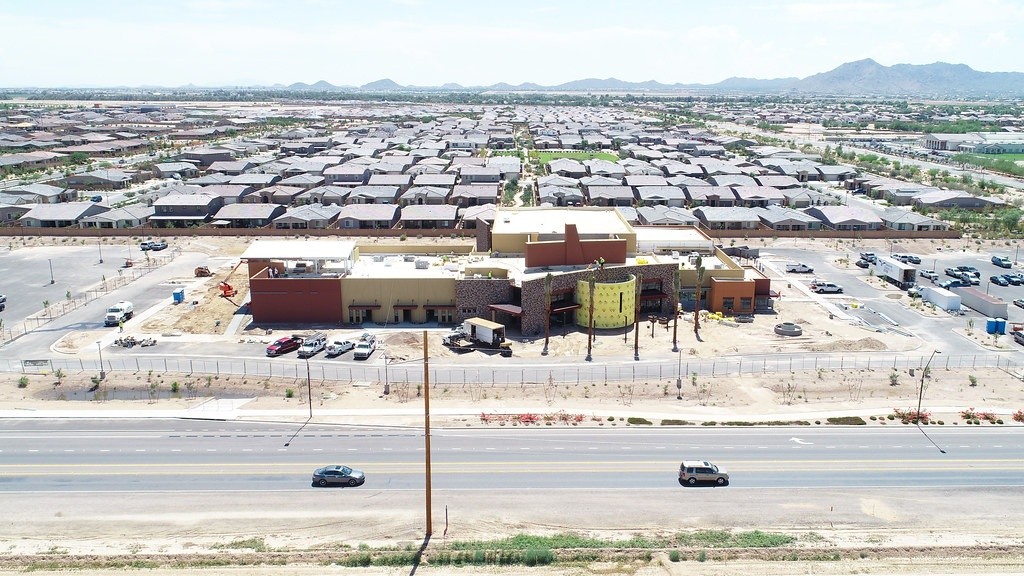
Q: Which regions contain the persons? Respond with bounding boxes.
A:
[119,317,123,332]
[269,267,288,278]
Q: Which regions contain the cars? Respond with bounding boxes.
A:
[311,464,366,489]
[266,336,300,356]
[856,252,1024,310]
[151,242,168,252]
[0,293,6,312]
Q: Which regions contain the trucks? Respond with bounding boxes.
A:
[297,332,327,357]
[353,332,377,359]
[458,316,507,347]
[104,301,134,327]
[91,196,102,202]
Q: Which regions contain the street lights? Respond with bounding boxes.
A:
[915,350,943,426]
[291,336,314,421]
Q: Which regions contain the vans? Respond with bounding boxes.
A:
[1014,330,1024,346]
[140,241,154,251]
[678,458,730,487]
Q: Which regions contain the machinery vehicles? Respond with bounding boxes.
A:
[194,265,215,278]
[219,281,238,296]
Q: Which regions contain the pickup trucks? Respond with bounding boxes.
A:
[785,262,815,273]
[325,339,356,356]
[810,281,844,294]
